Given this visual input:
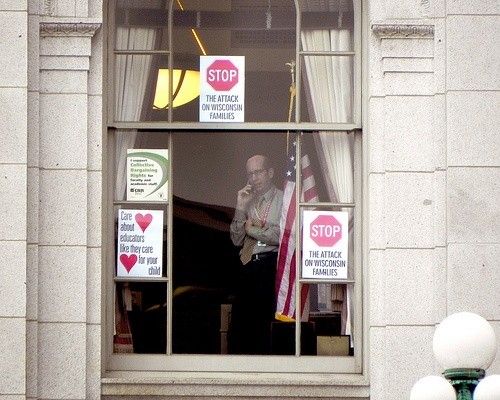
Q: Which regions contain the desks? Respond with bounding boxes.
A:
[305,311,341,357]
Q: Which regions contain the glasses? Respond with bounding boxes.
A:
[245,170,266,177]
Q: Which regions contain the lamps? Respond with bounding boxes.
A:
[152,0,208,112]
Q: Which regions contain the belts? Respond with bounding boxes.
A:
[251,252,279,261]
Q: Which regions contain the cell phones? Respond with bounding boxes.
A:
[246,179,254,195]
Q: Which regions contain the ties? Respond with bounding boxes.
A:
[256,197,265,211]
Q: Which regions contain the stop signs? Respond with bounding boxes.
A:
[199,55,247,126]
[303,210,346,281]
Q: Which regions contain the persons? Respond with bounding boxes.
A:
[229,154,300,356]
